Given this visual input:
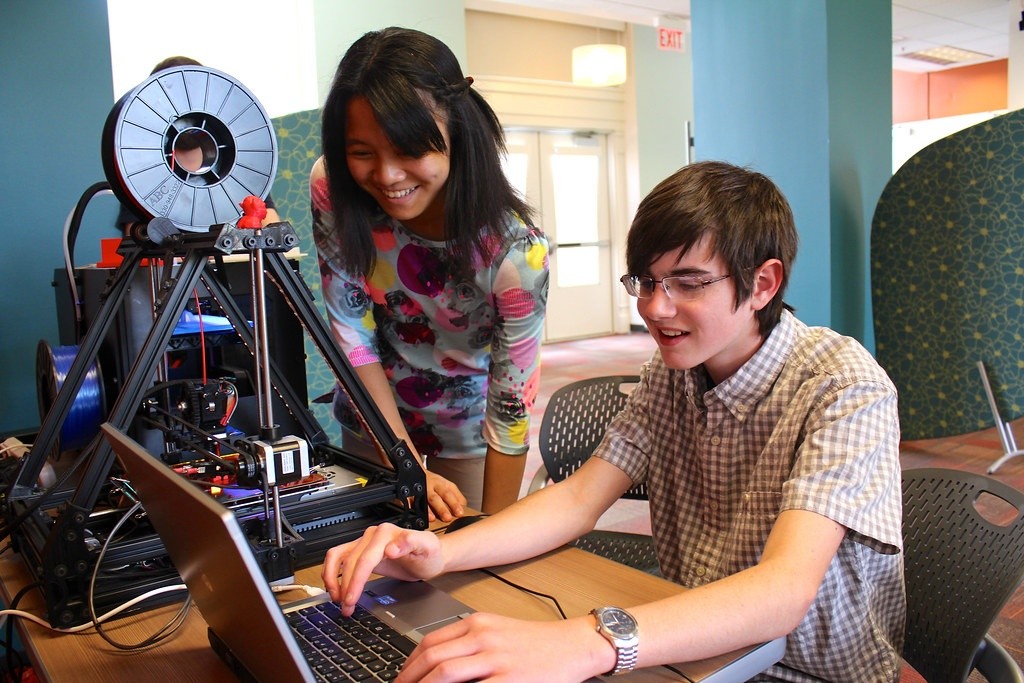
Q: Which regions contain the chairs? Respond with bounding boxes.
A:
[895,469,1024,683]
[526,375,658,573]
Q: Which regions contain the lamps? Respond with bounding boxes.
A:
[571,25,625,87]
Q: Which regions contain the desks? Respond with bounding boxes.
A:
[0,512,786,683]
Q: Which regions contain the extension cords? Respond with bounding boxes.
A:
[0,437,57,490]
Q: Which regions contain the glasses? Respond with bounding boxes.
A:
[620,266,757,302]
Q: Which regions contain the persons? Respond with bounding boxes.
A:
[310,25,550,521]
[321,162,907,683]
[112,55,280,230]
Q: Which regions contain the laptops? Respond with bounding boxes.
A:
[99,422,479,683]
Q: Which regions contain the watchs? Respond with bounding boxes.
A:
[590,606,638,679]
